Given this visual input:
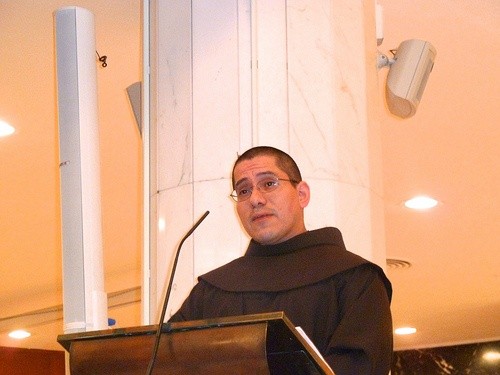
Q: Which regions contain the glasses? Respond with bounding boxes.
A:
[230,176,299,202]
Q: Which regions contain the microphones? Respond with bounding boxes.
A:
[146,211,210,375]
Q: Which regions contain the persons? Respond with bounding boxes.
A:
[167,145,393,375]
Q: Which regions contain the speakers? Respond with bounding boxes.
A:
[386,39,437,118]
